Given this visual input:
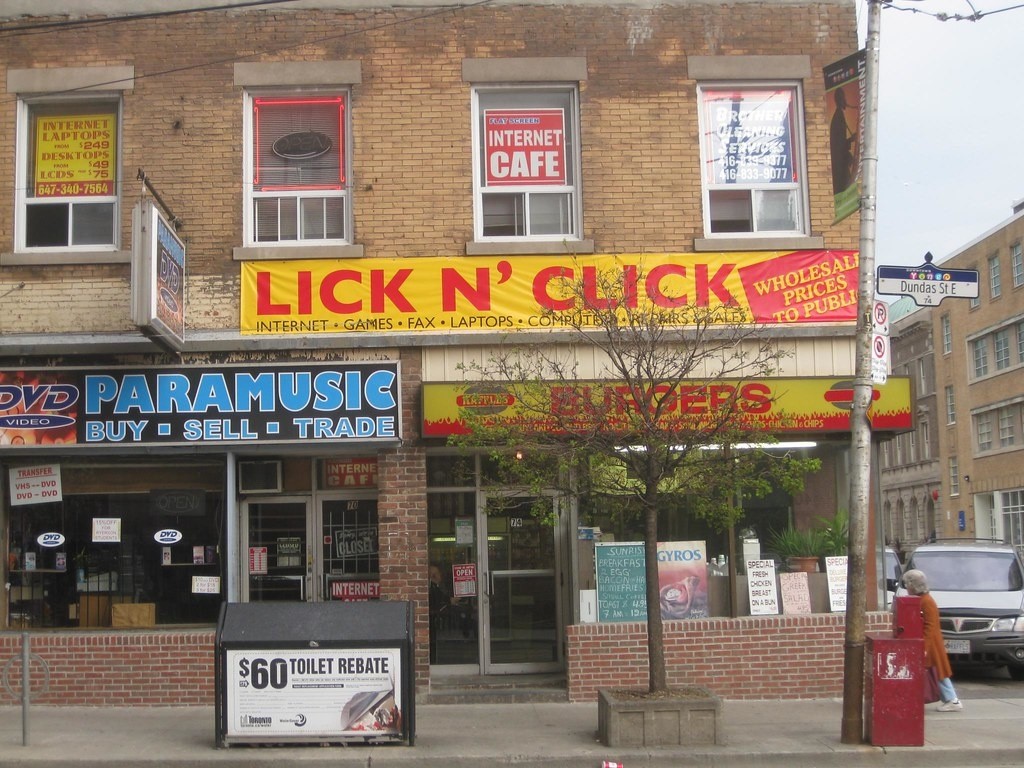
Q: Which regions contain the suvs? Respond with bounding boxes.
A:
[887,536,1024,680]
[872,547,902,610]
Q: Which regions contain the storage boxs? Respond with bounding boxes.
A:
[161,547,171,565]
[205,546,216,563]
[8,586,45,603]
[110,602,155,627]
[22,552,36,570]
[193,546,204,564]
[56,553,66,572]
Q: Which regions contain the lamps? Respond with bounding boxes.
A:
[614,441,818,454]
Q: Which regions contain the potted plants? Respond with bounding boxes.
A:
[764,523,824,574]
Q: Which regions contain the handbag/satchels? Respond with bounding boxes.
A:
[921,665,941,703]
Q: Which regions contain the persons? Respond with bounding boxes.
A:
[903,569,963,712]
[427,563,477,640]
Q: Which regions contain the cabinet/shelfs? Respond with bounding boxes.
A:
[78,593,140,627]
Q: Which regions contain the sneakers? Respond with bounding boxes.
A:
[936,699,963,711]
